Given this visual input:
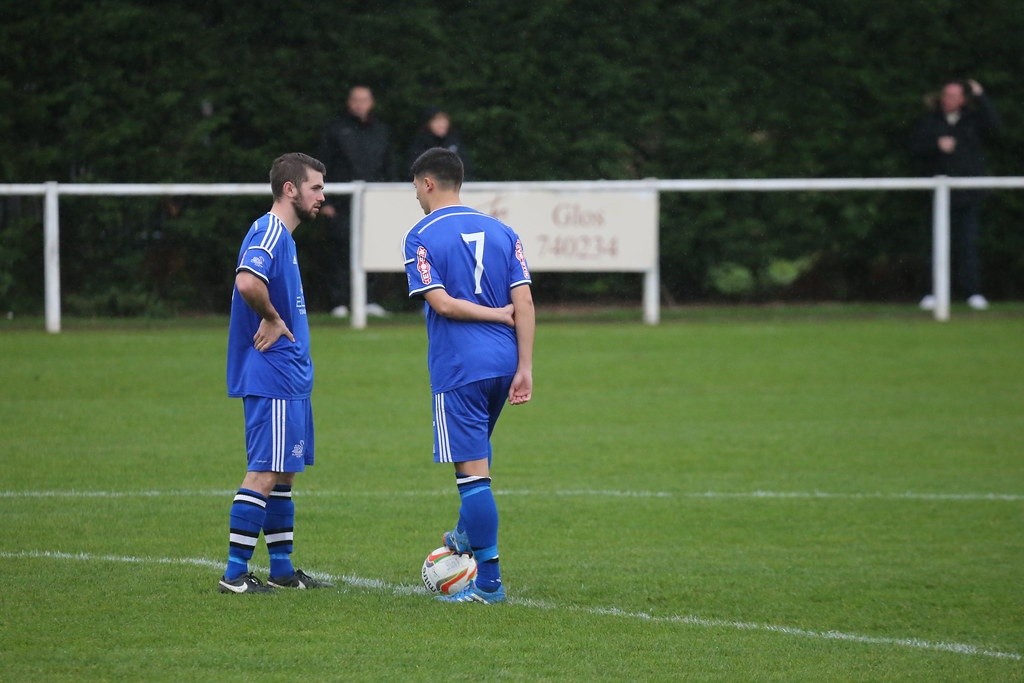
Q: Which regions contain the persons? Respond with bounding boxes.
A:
[403,111,482,181]
[318,85,398,316]
[218,152,332,594]
[908,73,1007,311]
[401,148,535,605]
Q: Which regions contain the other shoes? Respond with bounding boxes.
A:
[366,303,388,319]
[919,295,935,311]
[966,295,988,309]
[333,306,348,319]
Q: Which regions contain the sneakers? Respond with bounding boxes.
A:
[218,572,276,595]
[267,569,334,591]
[432,580,507,607]
[442,532,473,558]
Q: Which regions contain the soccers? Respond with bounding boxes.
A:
[421,545,478,596]
